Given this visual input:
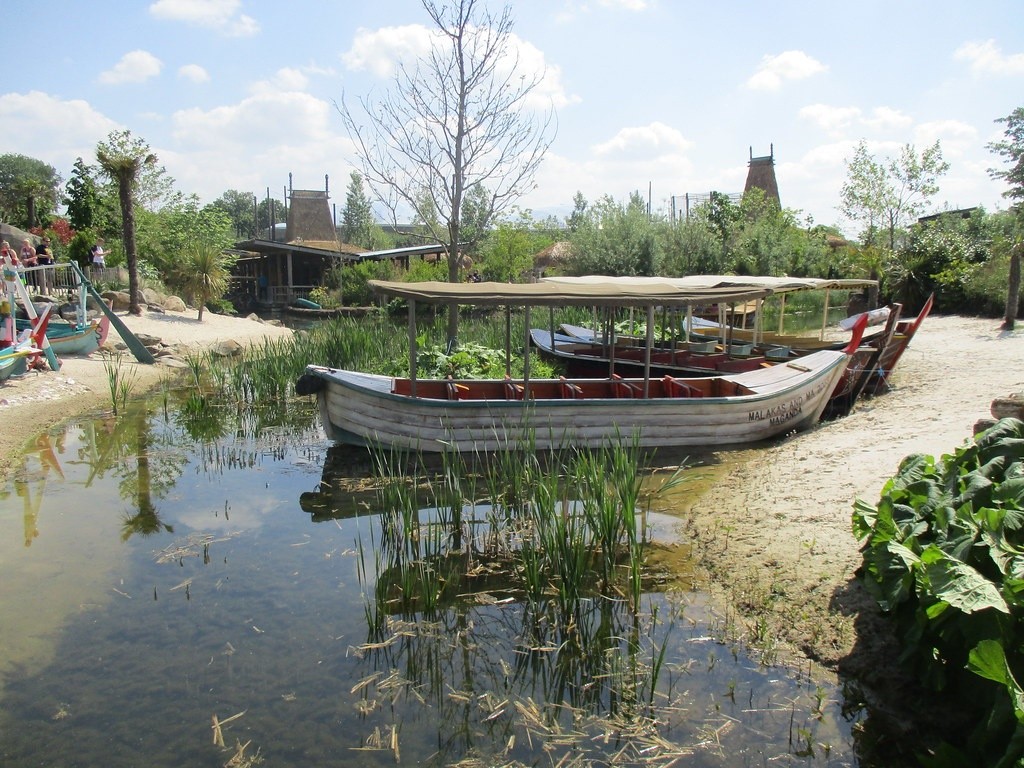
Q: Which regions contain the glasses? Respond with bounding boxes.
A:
[45,241,51,242]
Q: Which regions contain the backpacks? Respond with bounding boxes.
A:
[88,250,94,261]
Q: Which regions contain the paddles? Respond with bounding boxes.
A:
[67,258,156,365]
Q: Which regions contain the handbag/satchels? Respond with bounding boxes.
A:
[27,260,34,267]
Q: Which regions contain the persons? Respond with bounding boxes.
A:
[1,240,17,259]
[258,271,268,301]
[473,269,481,282]
[20,239,38,293]
[90,239,112,272]
[36,236,55,295]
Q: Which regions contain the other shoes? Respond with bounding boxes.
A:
[48,291,54,296]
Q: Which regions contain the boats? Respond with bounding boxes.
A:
[293,278,936,456]
[0,262,116,383]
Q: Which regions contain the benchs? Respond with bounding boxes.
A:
[503,375,523,399]
[559,375,583,399]
[632,340,646,347]
[689,340,718,352]
[764,346,791,357]
[447,375,464,400]
[726,343,755,355]
[610,374,643,399]
[574,344,626,357]
[605,348,664,361]
[654,339,678,349]
[663,375,703,398]
[715,356,766,373]
[640,350,692,364]
[676,354,724,369]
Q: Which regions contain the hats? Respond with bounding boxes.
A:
[24,238,30,242]
